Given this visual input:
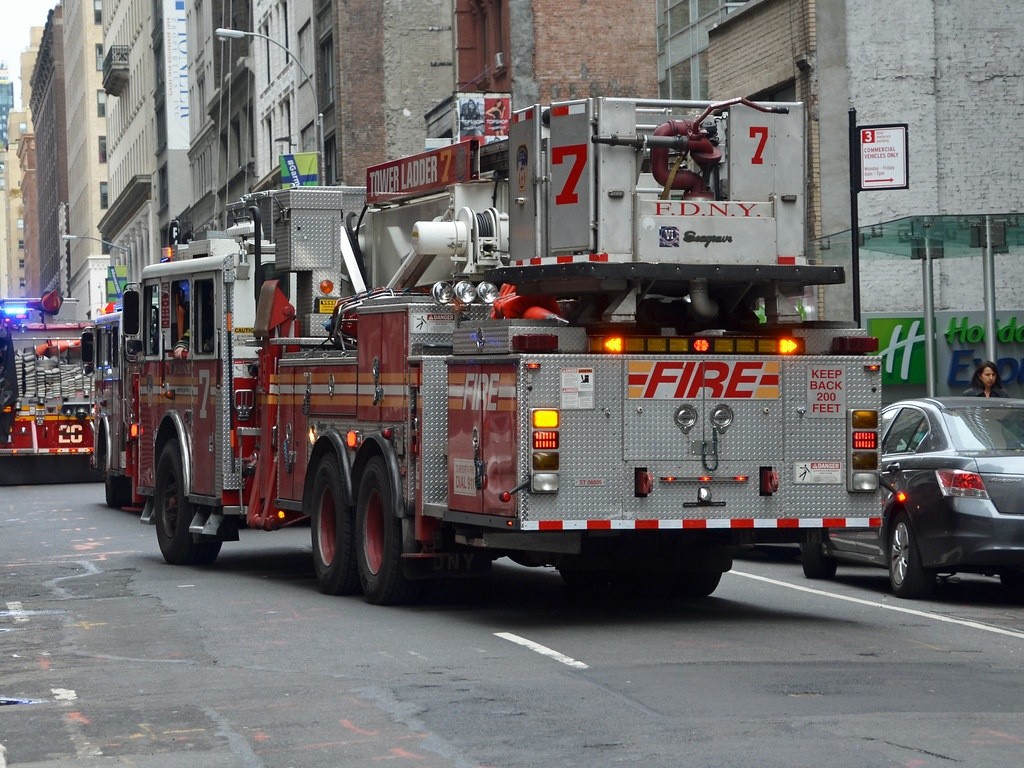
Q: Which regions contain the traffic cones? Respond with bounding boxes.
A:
[35,337,81,357]
[489,283,571,325]
[37,289,64,315]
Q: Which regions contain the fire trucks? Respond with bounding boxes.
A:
[123,94,887,605]
[81,311,143,509]
[0,296,103,481]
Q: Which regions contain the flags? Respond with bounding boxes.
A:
[281,152,318,188]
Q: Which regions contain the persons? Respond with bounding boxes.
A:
[173,330,190,359]
[460,99,484,136]
[963,361,1010,398]
[486,100,506,136]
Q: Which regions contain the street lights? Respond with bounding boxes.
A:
[215,28,323,187]
[61,235,131,287]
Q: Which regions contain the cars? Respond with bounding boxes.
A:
[801,396,1024,598]
